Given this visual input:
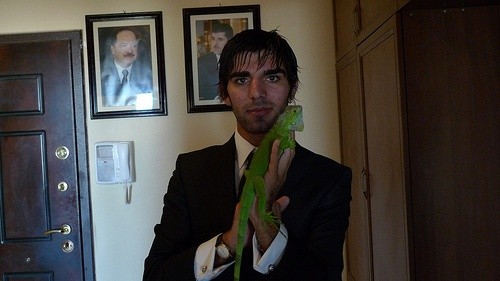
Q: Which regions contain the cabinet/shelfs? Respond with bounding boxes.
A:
[332,0,397,63]
[335,14,410,281]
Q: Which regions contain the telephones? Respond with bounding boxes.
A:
[94,140,136,205]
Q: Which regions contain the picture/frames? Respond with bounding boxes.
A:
[85,10,168,120]
[182,4,261,113]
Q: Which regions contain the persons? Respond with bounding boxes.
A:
[101,29,153,106]
[142,27,352,281]
[198,23,232,100]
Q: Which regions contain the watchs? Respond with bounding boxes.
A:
[215,234,235,262]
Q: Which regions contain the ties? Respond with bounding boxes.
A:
[236,150,254,200]
